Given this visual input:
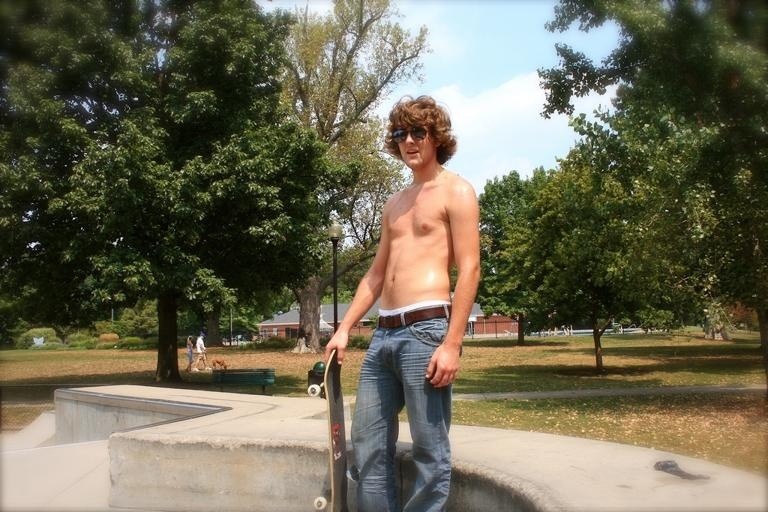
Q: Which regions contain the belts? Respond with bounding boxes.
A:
[376,305,453,330]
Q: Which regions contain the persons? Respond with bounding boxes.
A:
[322,93,484,512]
[185,335,194,371]
[194,331,209,371]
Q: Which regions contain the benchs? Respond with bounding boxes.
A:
[213,368,275,394]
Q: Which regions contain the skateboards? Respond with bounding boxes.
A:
[307,347,349,512]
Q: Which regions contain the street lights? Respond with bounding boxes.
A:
[329,221,342,334]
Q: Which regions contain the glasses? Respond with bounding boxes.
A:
[391,126,431,143]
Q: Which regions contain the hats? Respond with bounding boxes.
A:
[200,333,207,338]
